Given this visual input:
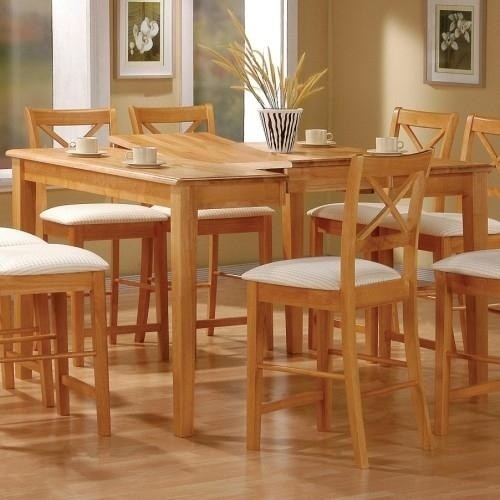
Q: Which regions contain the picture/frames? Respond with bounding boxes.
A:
[424,0,487,90]
[112,1,176,80]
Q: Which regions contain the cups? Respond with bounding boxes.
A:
[376,136,404,152]
[68,137,100,154]
[125,147,158,163]
[305,128,334,144]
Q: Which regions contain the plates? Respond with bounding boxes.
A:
[123,159,167,169]
[296,140,337,147]
[366,149,409,156]
[65,150,109,158]
